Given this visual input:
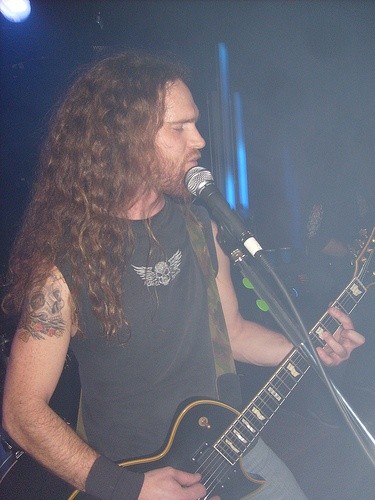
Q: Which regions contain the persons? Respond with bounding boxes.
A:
[1,51,365,500]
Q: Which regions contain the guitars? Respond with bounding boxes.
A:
[65,224,375,500]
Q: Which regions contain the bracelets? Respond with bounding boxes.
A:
[85,455,145,500]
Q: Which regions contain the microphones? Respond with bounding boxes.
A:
[183,165,265,259]
